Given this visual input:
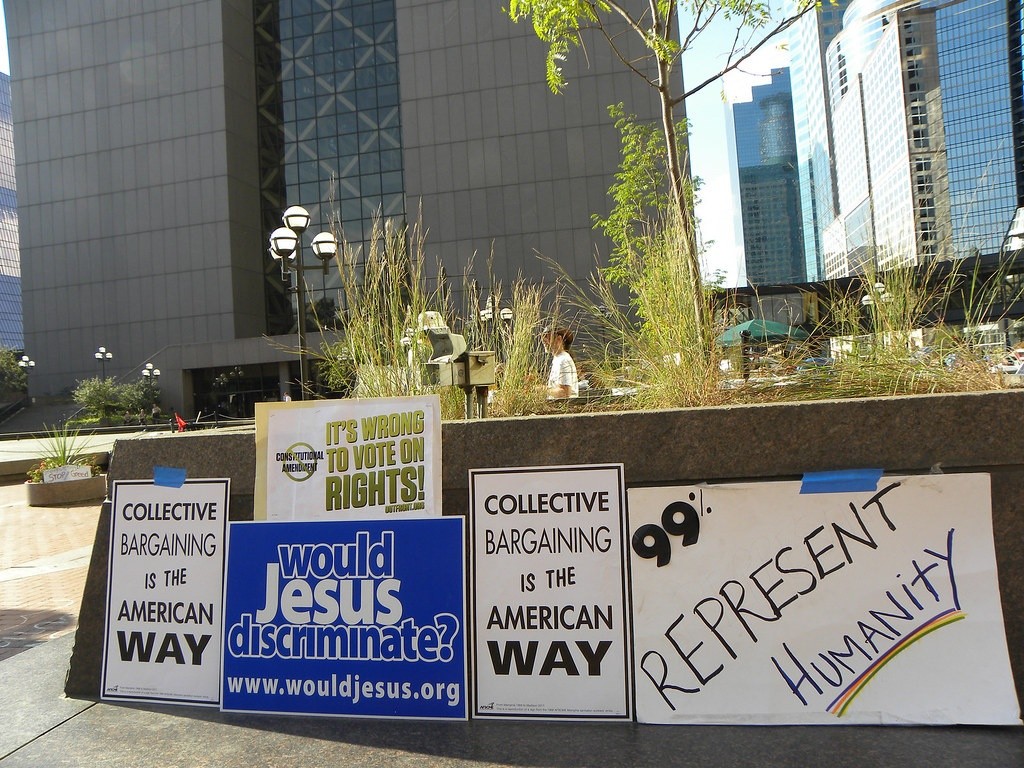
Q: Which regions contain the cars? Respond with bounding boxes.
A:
[904,343,1024,375]
[578,351,680,397]
[716,354,834,390]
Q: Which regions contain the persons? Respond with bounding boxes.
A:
[138,409,147,431]
[534,324,579,399]
[152,403,161,423]
[284,392,291,402]
[124,411,133,425]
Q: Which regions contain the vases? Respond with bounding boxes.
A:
[25,475,106,506]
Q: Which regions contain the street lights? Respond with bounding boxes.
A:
[95,346,112,381]
[18,355,36,385]
[479,293,513,362]
[213,366,245,419]
[861,283,895,349]
[268,205,338,400]
[141,363,161,408]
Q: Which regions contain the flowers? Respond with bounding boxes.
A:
[26,422,105,484]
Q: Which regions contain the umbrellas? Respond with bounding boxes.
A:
[715,318,808,354]
